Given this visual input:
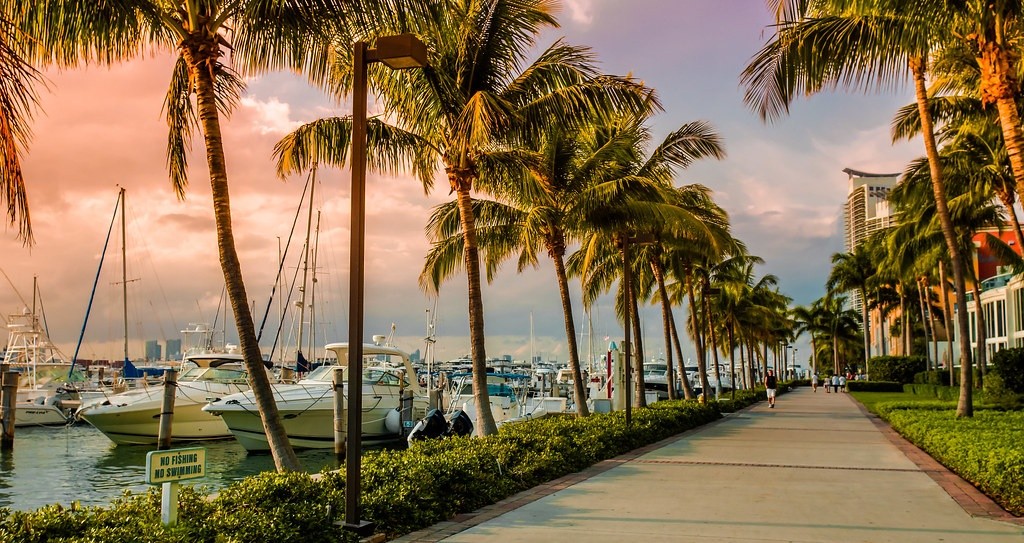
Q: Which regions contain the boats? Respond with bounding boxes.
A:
[0,164,739,452]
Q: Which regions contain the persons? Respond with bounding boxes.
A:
[824,374,846,393]
[765,370,777,408]
[812,373,818,392]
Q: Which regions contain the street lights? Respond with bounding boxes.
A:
[702,288,721,404]
[343,29,428,535]
